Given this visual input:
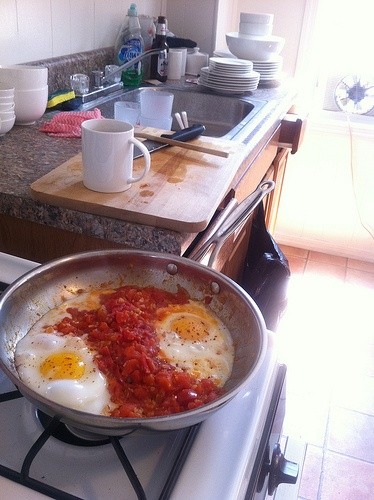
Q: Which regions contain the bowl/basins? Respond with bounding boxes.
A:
[0,65,48,90]
[0,85,48,125]
[238,12,273,41]
[0,87,16,136]
[225,32,285,61]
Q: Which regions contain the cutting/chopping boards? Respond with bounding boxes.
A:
[29,125,246,233]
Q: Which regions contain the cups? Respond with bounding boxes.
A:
[169,47,187,79]
[81,119,151,193]
[139,90,174,131]
[114,100,141,125]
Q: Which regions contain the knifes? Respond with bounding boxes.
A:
[133,124,206,160]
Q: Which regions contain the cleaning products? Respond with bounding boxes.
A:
[112,3,146,89]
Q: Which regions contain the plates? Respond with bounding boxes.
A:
[334,71,374,115]
[197,48,282,94]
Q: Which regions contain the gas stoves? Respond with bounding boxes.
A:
[0,251,300,500]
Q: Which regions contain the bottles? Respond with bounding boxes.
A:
[150,16,170,82]
[114,3,145,87]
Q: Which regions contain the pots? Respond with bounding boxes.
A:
[0,180,275,435]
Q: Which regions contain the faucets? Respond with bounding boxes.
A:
[91,48,168,91]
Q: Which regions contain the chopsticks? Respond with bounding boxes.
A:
[175,111,189,130]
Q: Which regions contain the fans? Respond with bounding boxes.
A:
[322,69,374,117]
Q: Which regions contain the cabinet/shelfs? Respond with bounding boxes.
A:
[186,105,310,285]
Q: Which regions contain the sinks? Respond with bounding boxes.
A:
[80,83,269,140]
